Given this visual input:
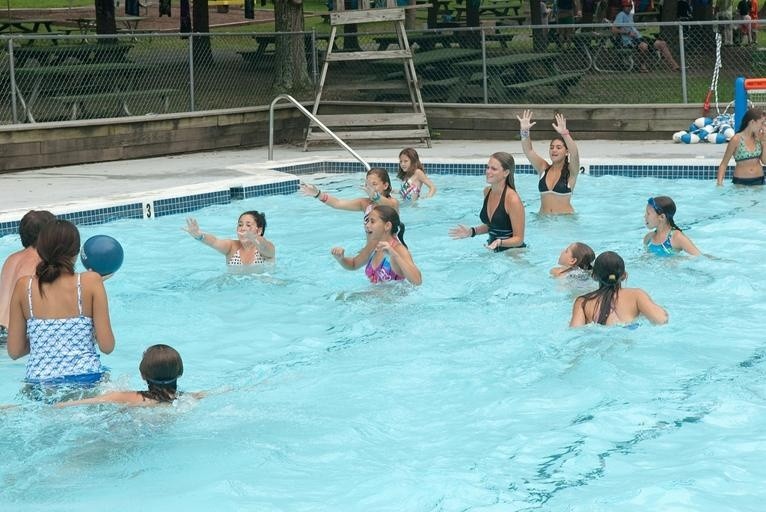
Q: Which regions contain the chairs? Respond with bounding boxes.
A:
[592,21,662,79]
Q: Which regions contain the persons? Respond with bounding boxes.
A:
[0,210,58,346]
[551,242,594,292]
[516,110,579,215]
[6,343,243,432]
[716,110,766,188]
[448,151,528,255]
[178,211,275,271]
[6,219,115,406]
[298,148,435,301]
[643,196,703,260]
[528,0,758,75]
[569,250,669,331]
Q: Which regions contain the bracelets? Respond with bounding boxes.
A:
[559,128,569,135]
[519,129,530,136]
[470,226,475,238]
[194,232,204,240]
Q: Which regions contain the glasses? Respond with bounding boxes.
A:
[648,198,664,213]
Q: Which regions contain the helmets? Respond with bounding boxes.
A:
[621,0,632,6]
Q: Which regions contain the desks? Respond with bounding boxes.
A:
[369,47,480,101]
[253,33,342,69]
[444,51,569,104]
[453,2,524,27]
[373,35,466,50]
[1,12,177,109]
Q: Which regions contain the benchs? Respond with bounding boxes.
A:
[422,71,514,89]
[505,72,580,92]
[265,47,361,58]
[237,50,276,61]
[449,16,526,26]
[449,35,513,41]
